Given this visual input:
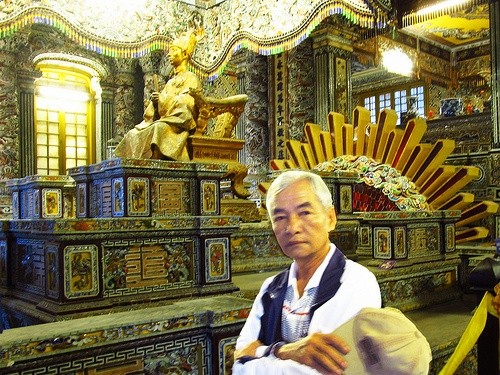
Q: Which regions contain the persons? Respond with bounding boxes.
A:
[230,170,381,375]
[115,27,204,161]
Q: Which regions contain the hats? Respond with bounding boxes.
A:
[329,307,433,374]
